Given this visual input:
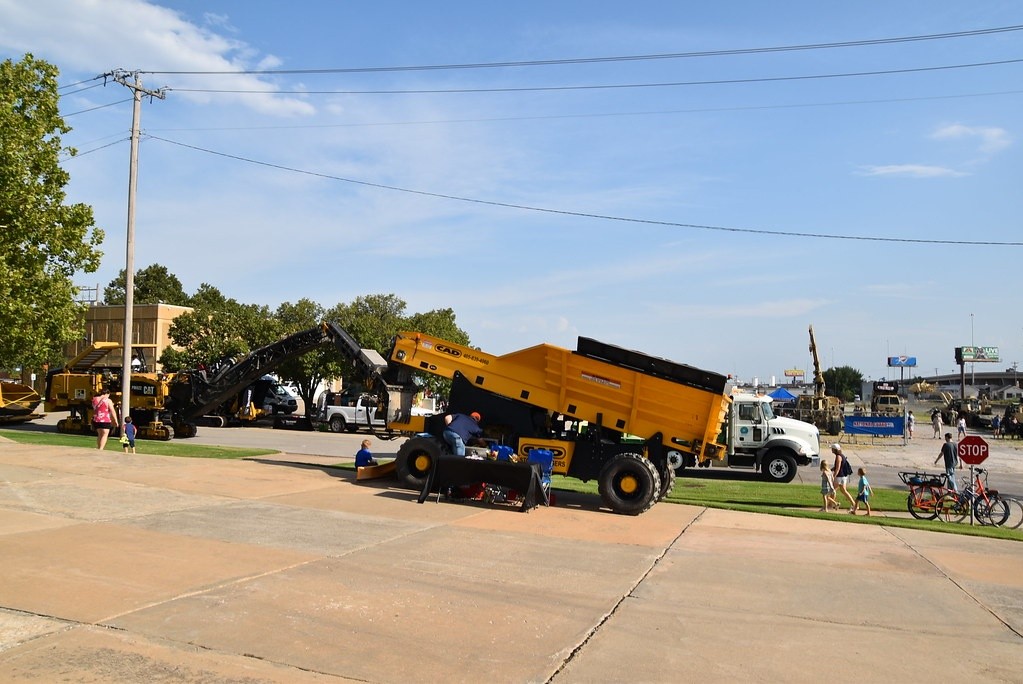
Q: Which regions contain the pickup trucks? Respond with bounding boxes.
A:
[324,393,436,433]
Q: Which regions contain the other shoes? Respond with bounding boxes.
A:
[848,511,855,516]
[834,503,840,511]
[818,509,829,513]
[864,513,871,516]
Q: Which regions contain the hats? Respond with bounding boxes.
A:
[470,412,481,422]
[831,444,841,451]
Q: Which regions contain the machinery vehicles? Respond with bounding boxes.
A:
[788,325,842,435]
[43,340,414,442]
[910,382,981,424]
[976,394,992,414]
[0,381,46,425]
[1008,401,1023,424]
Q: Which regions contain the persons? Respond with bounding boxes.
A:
[91,388,119,450]
[442,411,487,457]
[131,355,141,372]
[853,405,915,440]
[931,406,959,440]
[819,443,874,517]
[354,439,378,472]
[956,415,967,442]
[122,416,137,454]
[933,432,963,499]
[992,414,1006,439]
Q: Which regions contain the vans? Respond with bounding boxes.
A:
[260,375,298,415]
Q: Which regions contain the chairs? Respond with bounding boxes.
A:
[474,445,514,504]
[514,449,553,508]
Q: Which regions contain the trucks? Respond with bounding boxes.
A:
[871,381,902,416]
[666,392,823,483]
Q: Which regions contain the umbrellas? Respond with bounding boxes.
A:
[767,387,797,400]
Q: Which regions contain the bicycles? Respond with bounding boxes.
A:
[898,466,1023,529]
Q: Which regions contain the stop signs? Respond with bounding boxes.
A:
[957,435,989,465]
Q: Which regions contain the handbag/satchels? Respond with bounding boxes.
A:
[91,418,94,431]
[839,455,853,477]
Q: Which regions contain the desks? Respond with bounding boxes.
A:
[418,454,549,513]
